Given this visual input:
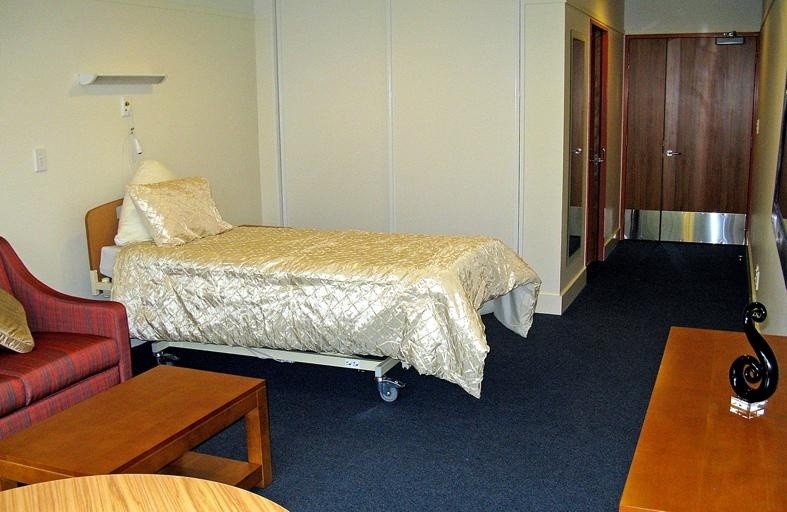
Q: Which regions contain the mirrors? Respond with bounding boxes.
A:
[566,30,588,265]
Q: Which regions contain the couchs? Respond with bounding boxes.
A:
[0,236,131,440]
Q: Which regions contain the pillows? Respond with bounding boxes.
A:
[127,177,232,247]
[114,161,174,245]
[0,288,34,353]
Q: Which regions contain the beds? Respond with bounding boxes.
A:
[85,199,540,402]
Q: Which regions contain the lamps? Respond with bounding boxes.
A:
[129,126,143,155]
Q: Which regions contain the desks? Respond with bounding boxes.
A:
[619,327,787,512]
[0,474,289,512]
